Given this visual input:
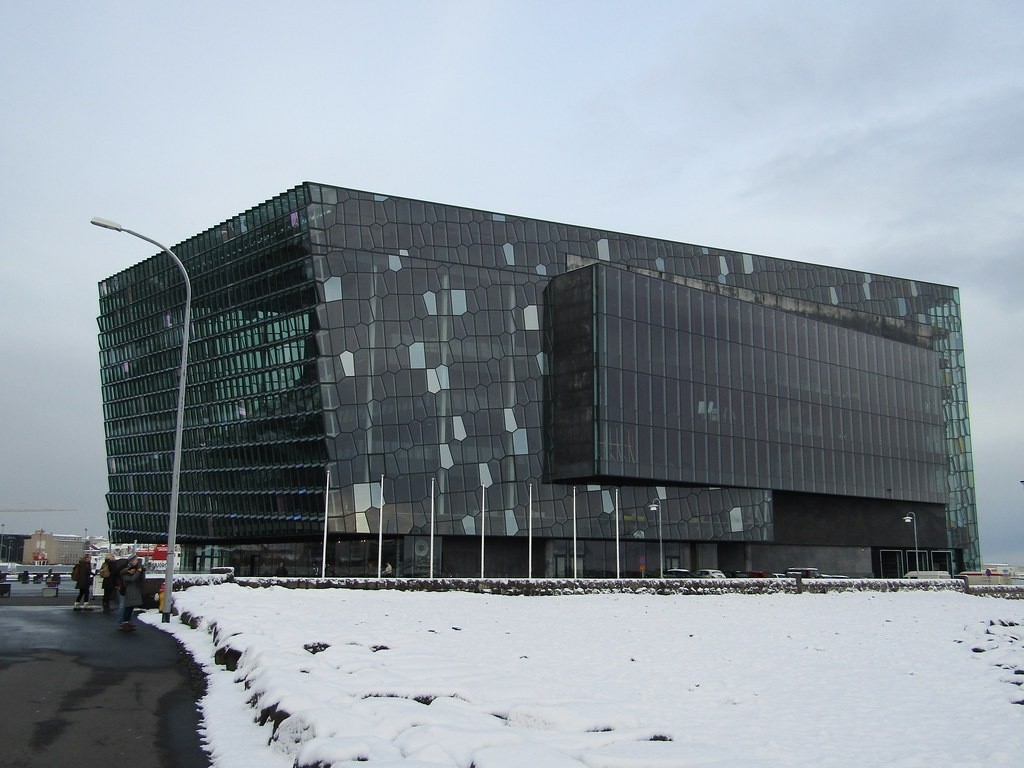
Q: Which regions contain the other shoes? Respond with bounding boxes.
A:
[103,607,113,612]
[84,607,93,611]
[118,621,138,630]
[74,607,83,611]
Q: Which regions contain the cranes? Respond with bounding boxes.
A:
[0,508,78,512]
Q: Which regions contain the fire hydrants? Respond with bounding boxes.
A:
[154,582,165,614]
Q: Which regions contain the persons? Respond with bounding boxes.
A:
[75,554,95,610]
[328,563,333,576]
[385,561,392,578]
[313,563,319,576]
[275,563,288,577]
[102,555,121,611]
[117,556,143,630]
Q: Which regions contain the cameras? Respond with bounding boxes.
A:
[140,568,146,574]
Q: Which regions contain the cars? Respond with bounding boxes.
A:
[663,567,820,578]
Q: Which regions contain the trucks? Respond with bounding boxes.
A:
[85,544,181,571]
[904,571,951,579]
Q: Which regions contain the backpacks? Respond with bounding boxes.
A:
[118,577,126,595]
[100,562,110,577]
[71,565,80,581]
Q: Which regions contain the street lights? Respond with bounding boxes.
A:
[902,512,920,578]
[0,524,5,560]
[90,216,191,622]
[648,498,663,578]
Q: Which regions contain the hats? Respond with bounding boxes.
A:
[128,554,136,562]
